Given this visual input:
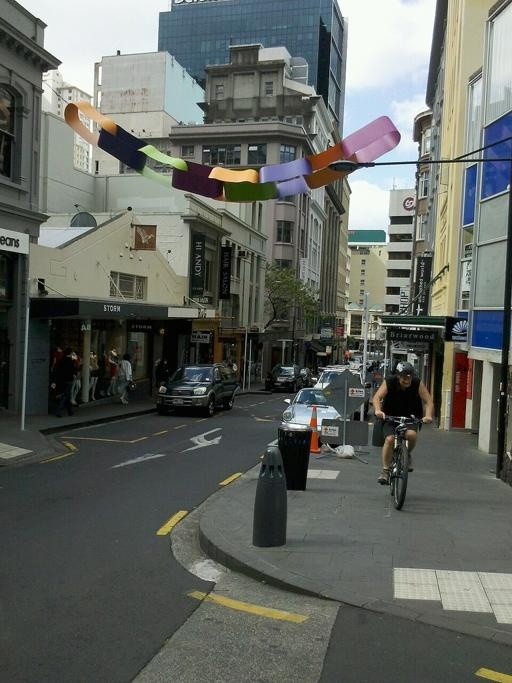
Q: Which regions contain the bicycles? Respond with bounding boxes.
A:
[375,414,432,510]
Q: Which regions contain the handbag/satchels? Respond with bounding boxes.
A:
[128,382,136,392]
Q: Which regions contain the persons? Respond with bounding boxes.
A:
[68,352,82,406]
[345,350,350,364]
[155,359,169,388]
[373,361,433,485]
[52,347,64,368]
[89,352,100,401]
[117,354,132,405]
[106,348,119,396]
[51,348,74,416]
[368,359,386,389]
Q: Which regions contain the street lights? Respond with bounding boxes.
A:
[328,160,511,478]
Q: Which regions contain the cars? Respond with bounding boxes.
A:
[156,363,241,417]
[265,363,312,394]
[278,355,390,452]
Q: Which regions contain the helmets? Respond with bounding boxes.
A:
[396,361,414,376]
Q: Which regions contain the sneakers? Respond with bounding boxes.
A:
[408,453,413,472]
[377,467,392,483]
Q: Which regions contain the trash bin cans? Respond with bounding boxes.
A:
[278,426,312,490]
